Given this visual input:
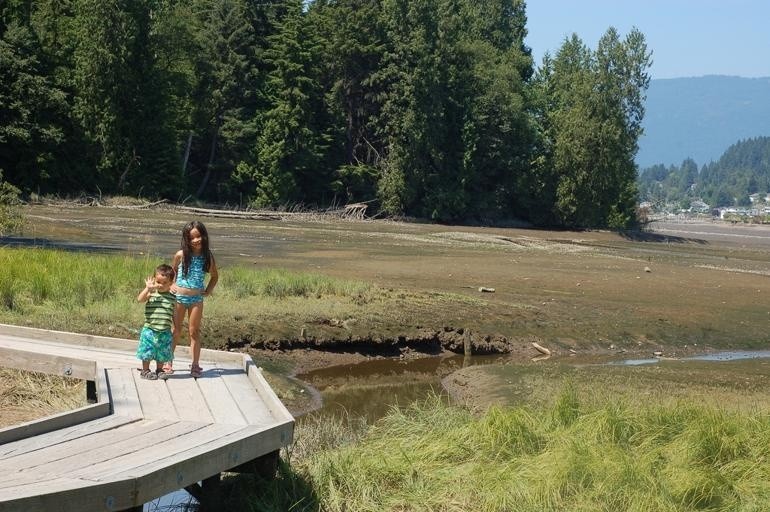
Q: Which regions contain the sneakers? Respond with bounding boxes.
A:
[164,363,172,373]
[192,363,202,377]
[141,369,157,380]
[156,369,167,380]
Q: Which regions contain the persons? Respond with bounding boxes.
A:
[137,264,180,380]
[162,220,218,378]
